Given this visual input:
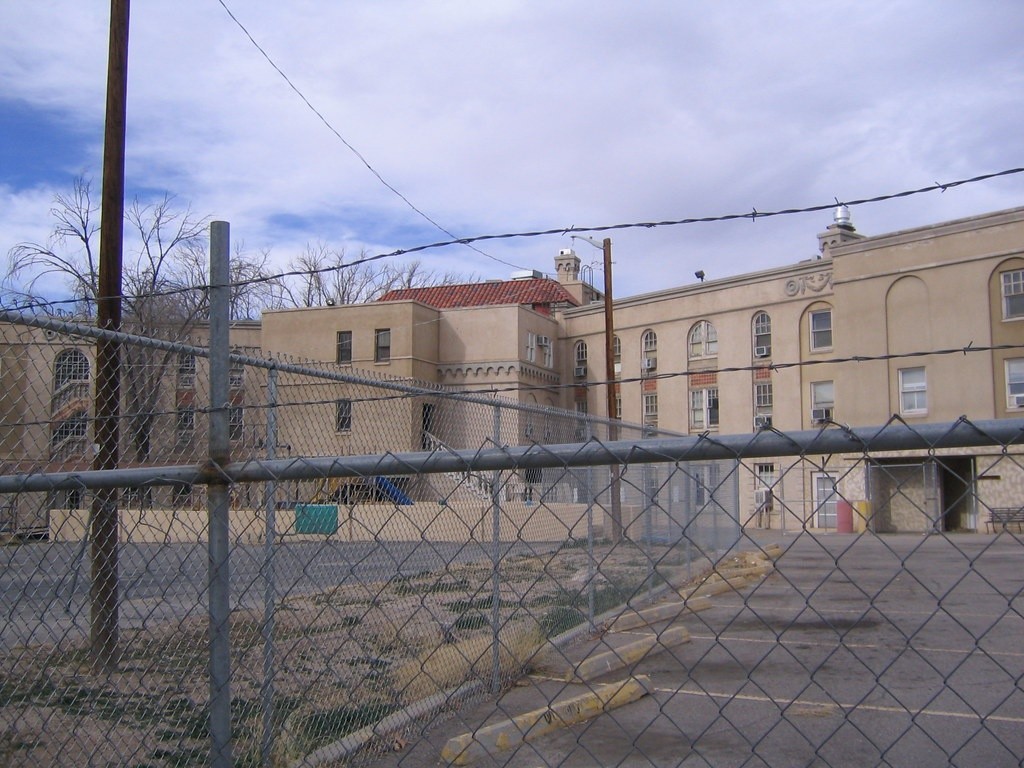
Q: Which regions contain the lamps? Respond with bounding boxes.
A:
[326,298,335,306]
[695,270,704,282]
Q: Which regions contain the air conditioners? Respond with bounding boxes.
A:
[754,489,772,507]
[574,367,586,377]
[812,408,832,421]
[754,346,769,357]
[642,357,656,370]
[538,336,550,347]
[574,428,586,439]
[753,416,770,428]
[1015,395,1024,407]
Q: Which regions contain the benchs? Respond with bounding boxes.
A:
[984,507,1024,533]
